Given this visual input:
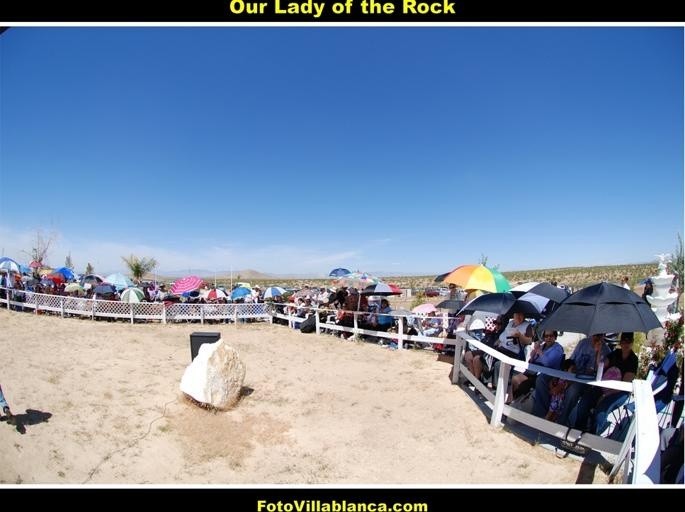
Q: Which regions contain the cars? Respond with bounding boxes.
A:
[285,286,300,292]
[424,287,469,301]
[218,285,226,291]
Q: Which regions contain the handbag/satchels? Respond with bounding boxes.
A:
[299,313,316,332]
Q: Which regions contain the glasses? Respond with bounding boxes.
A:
[543,333,556,338]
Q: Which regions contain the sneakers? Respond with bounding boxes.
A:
[4,406,11,418]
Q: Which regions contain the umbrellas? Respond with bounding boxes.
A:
[434,271,452,283]
[436,299,465,310]
[434,264,511,294]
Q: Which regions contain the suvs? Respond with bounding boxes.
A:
[234,282,252,289]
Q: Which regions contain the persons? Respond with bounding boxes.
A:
[454,277,663,456]
[0,386,16,424]
[0,254,443,353]
[445,310,464,356]
[446,284,460,332]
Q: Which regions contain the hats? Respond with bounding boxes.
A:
[493,316,501,325]
[601,366,622,395]
[619,332,634,342]
